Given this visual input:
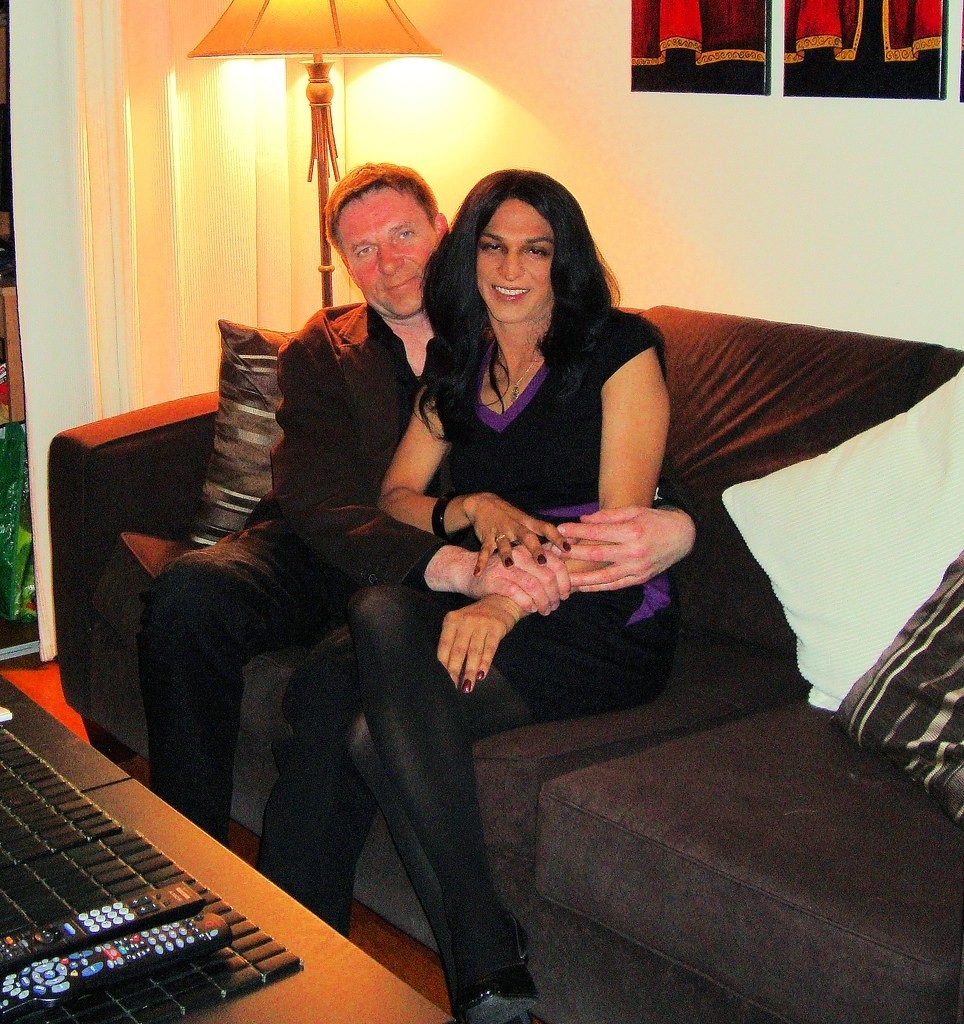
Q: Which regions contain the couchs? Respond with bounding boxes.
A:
[42,304,964,1023]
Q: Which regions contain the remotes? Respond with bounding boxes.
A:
[0,882,205,976]
[0,912,234,1023]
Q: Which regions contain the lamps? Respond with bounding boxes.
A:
[183,1,445,309]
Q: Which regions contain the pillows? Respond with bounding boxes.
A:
[718,365,964,711]
[828,550,964,829]
[177,315,306,552]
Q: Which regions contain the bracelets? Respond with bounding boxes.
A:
[431,490,468,547]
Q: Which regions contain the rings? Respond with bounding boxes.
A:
[494,532,507,542]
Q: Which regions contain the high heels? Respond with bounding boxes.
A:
[444,911,536,1024]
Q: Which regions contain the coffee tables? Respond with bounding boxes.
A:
[2,670,464,1023]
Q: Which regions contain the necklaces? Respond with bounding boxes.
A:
[498,346,544,404]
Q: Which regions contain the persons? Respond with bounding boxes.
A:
[131,159,699,940]
[336,168,681,1023]
